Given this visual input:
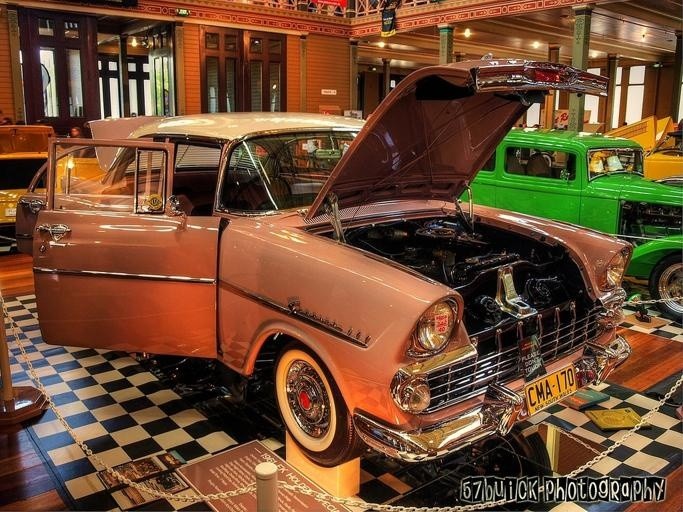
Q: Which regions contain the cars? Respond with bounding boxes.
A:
[0,59,683,466]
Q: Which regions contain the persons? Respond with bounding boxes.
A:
[70,126,87,158]
[0,109,52,138]
